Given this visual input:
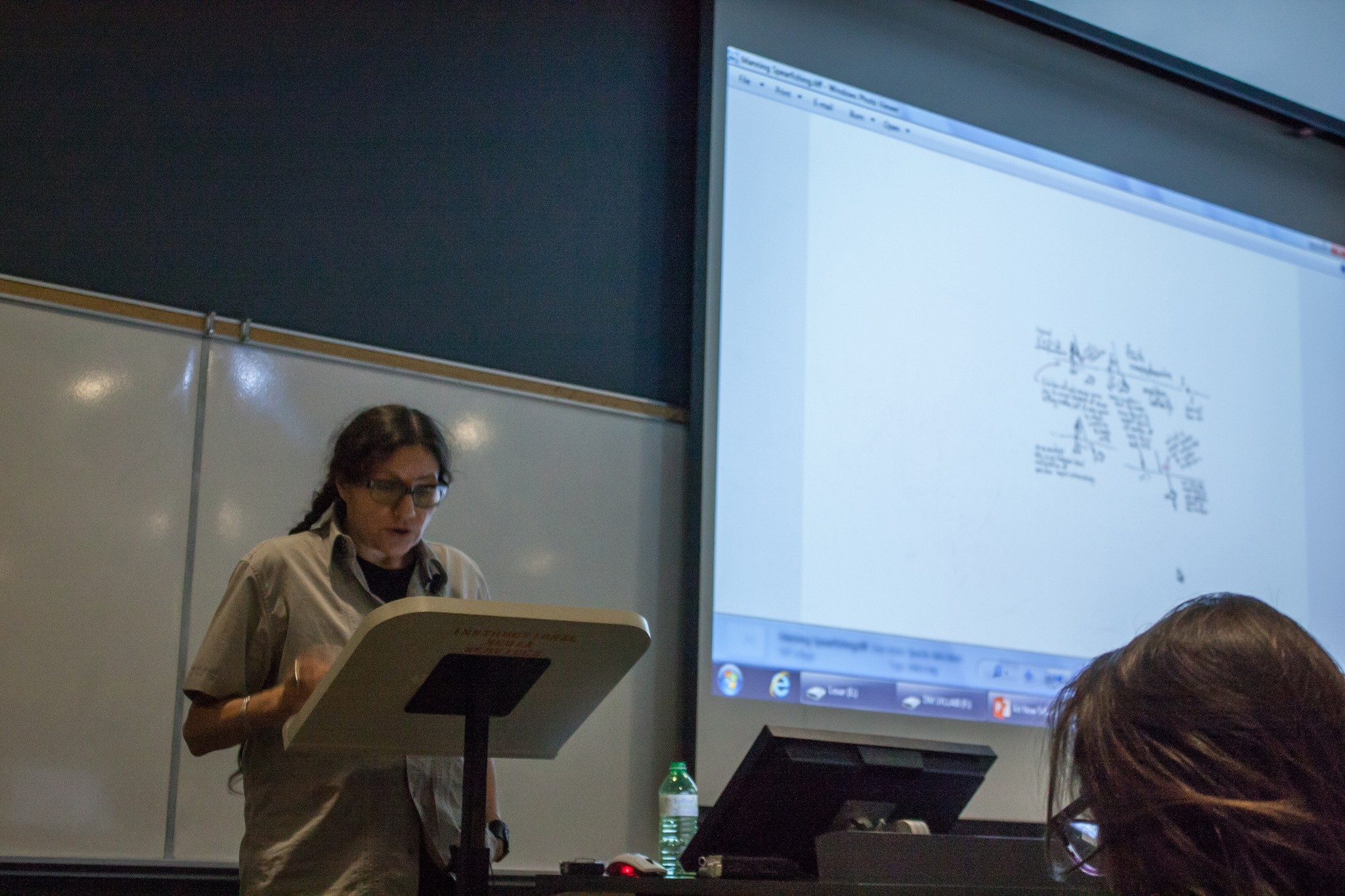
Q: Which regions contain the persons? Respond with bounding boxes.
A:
[181,403,511,896]
[1041,591,1345,896]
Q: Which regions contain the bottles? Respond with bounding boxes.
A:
[658,762,698,879]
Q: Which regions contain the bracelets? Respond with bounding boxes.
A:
[240,696,261,737]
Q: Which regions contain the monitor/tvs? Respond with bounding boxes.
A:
[681,725,998,879]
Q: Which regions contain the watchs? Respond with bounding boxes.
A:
[487,819,510,863]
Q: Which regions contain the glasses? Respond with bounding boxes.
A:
[1047,780,1121,877]
[353,478,449,508]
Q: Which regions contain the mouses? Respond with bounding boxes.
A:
[604,851,667,879]
